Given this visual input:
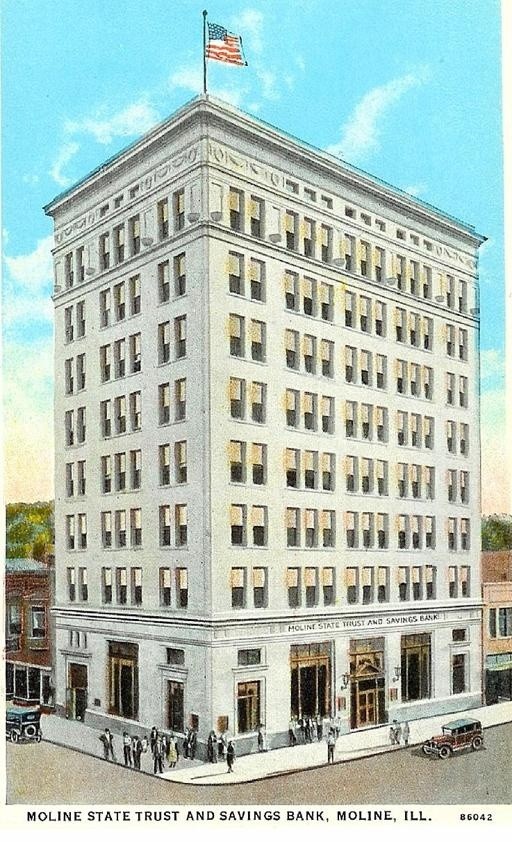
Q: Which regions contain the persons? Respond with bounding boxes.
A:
[254,722,269,752]
[94,725,236,778]
[388,719,411,748]
[288,713,344,766]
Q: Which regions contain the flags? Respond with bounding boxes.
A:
[204,20,247,66]
